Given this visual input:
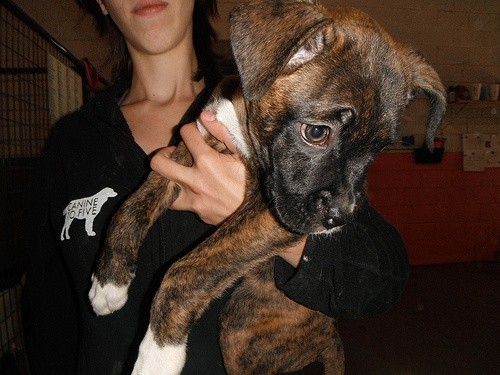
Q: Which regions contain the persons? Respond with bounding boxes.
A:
[0,0,421,375]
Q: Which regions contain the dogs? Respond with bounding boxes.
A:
[88,0,446,375]
